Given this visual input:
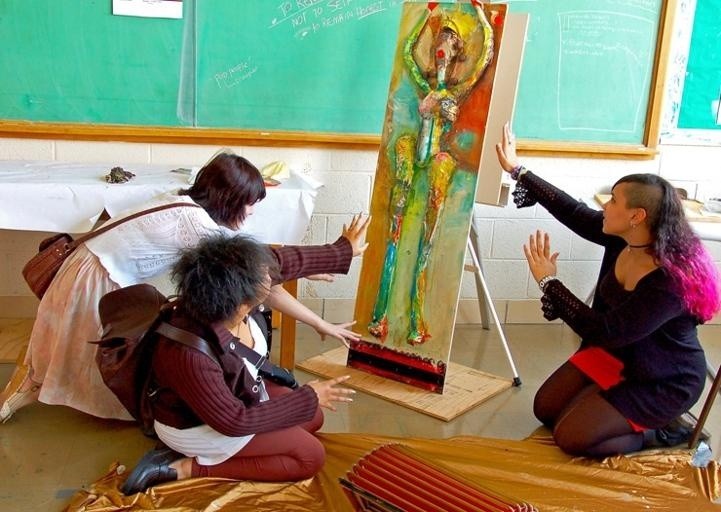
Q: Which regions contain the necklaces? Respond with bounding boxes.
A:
[626,241,652,254]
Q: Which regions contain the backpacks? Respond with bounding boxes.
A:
[88,283,222,439]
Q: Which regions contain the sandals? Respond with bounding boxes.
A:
[0,344,45,424]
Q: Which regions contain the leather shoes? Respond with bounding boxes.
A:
[120,445,188,497]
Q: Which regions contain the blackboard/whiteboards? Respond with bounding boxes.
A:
[0,0,677,160]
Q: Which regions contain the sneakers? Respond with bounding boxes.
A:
[655,412,713,449]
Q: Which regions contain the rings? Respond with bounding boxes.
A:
[509,140,512,145]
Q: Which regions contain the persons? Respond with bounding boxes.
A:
[87,212,372,496]
[1,148,365,423]
[496,122,721,461]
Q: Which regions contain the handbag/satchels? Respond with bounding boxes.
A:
[21,231,77,301]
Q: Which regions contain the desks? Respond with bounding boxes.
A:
[1,162,324,371]
[583,193,721,393]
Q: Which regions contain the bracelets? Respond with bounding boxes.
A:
[510,164,524,180]
[539,275,551,293]
